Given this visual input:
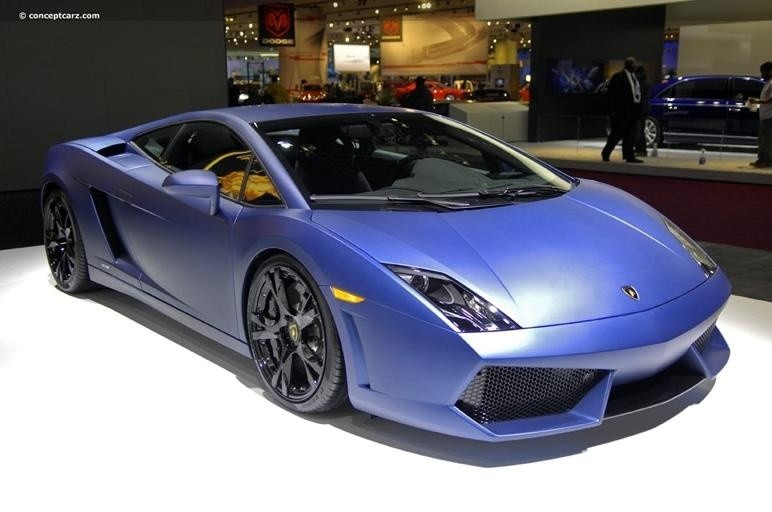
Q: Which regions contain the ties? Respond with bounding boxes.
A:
[631,73,639,100]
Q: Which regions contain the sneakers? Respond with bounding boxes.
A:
[750,160,772,168]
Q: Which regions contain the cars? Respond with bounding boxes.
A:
[643,75,764,147]
[229,78,532,109]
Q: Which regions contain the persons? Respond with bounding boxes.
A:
[407,77,432,113]
[746,61,772,168]
[227,77,241,107]
[630,62,647,158]
[595,54,643,162]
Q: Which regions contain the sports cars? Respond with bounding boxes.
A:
[37,101,738,444]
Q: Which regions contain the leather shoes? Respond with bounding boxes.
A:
[626,158,643,163]
[601,152,610,162]
